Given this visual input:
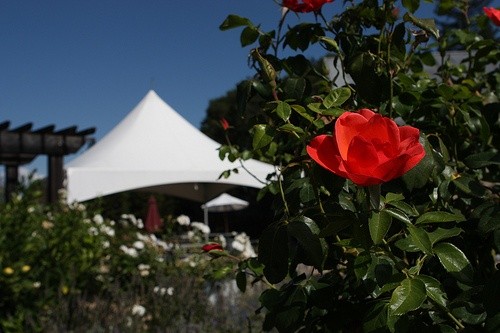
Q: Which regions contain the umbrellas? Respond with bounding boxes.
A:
[202,192,250,235]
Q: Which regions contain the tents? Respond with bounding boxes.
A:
[63,89,306,206]
